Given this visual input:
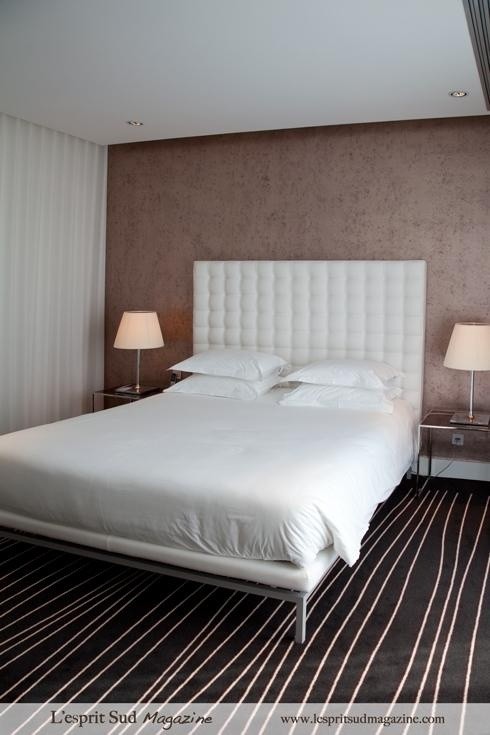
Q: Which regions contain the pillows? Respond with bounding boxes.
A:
[165,347,406,415]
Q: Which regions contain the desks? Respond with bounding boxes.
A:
[417,409,490,497]
[92,384,168,413]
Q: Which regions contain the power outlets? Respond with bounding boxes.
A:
[452,433,464,446]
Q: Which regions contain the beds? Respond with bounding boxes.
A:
[1,260,427,643]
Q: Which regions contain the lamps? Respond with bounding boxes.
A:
[443,322,490,427]
[113,311,165,395]
